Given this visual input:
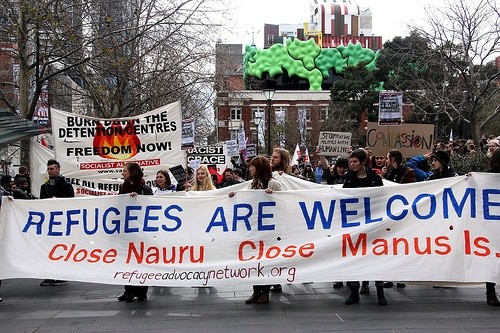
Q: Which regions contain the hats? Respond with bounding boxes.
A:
[431,152,450,166]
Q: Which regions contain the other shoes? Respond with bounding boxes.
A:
[377,288,390,305]
[344,290,360,305]
[39,279,55,286]
[53,279,69,286]
[397,283,406,288]
[245,291,259,304]
[269,286,283,293]
[360,285,370,294]
[485,284,500,306]
[384,282,394,288]
[333,282,343,288]
[118,289,134,301]
[133,294,148,301]
[258,292,270,303]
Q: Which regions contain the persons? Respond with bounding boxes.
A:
[407,135,500,307]
[40,159,74,285]
[0,166,36,200]
[270,148,290,292]
[294,146,412,305]
[155,151,252,193]
[117,163,153,302]
[229,156,281,303]
